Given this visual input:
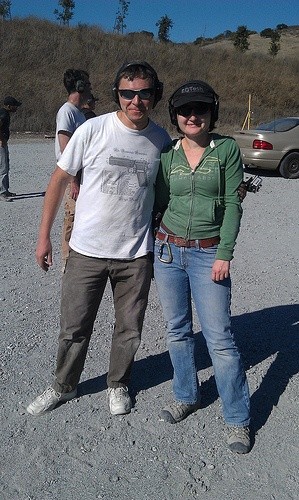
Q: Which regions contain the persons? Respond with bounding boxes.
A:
[26,61,247,414]
[153,80,252,454]
[0,96,22,202]
[54,68,92,259]
[82,94,99,119]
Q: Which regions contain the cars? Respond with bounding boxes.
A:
[233,117,299,179]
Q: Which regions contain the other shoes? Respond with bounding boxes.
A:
[0,194,13,201]
[5,191,16,197]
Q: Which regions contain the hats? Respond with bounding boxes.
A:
[88,94,100,101]
[4,96,22,106]
[171,80,215,107]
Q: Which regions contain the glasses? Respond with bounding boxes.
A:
[177,106,209,117]
[117,88,156,100]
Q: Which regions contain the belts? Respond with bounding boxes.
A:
[156,221,220,249]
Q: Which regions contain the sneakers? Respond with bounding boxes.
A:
[27,385,75,416]
[227,424,252,454]
[107,386,132,415]
[161,400,199,424]
